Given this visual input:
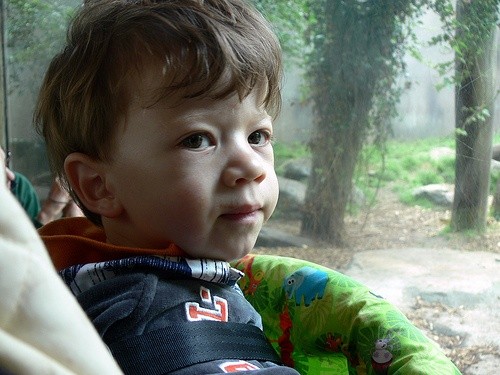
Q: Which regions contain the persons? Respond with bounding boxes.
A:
[34,0,308,373]
